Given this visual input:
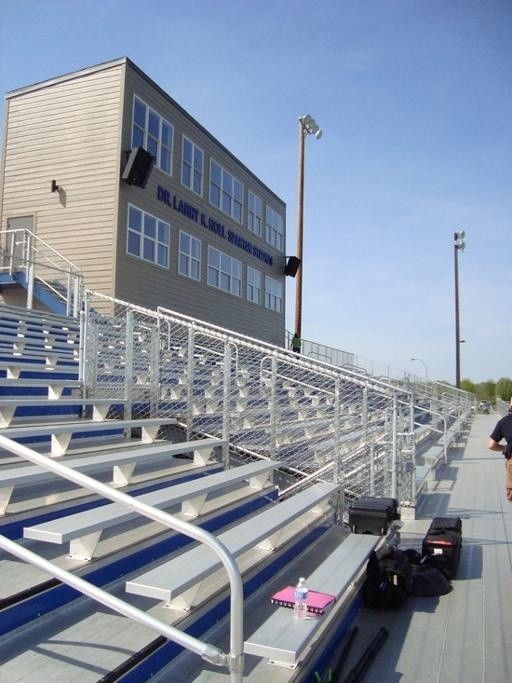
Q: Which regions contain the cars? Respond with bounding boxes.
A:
[479,400,488,407]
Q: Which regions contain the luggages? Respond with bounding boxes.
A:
[349,495,401,537]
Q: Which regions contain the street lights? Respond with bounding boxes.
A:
[293,115,323,355]
[454,231,467,384]
[411,358,428,391]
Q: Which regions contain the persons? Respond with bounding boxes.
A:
[291,333,301,360]
[488,405,512,503]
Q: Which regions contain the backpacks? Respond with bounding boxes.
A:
[360,545,414,612]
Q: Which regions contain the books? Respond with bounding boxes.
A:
[270,585,336,614]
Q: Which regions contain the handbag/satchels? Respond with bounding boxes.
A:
[423,515,468,581]
[404,547,453,597]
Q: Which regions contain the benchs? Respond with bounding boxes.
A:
[1,300,474,683]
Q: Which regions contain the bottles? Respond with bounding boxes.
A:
[293,577,308,621]
